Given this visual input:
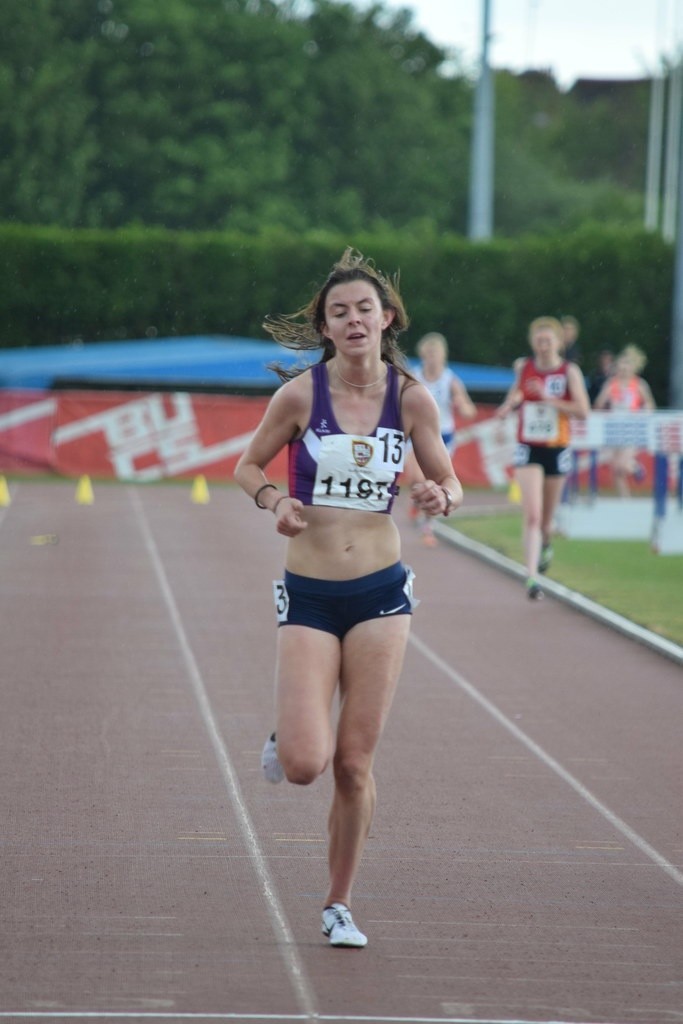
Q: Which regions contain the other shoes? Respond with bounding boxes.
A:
[538,544,555,572]
[527,580,542,599]
[421,528,436,547]
[407,503,419,518]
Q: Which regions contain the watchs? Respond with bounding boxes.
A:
[441,486,452,517]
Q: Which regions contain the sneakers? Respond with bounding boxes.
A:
[320,902,368,946]
[263,731,285,783]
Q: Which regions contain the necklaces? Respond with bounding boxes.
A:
[332,355,388,389]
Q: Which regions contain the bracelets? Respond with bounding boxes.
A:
[272,495,291,514]
[254,484,276,510]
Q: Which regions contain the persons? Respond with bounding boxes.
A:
[230,261,467,947]
[559,314,661,499]
[495,314,593,601]
[406,331,478,548]
